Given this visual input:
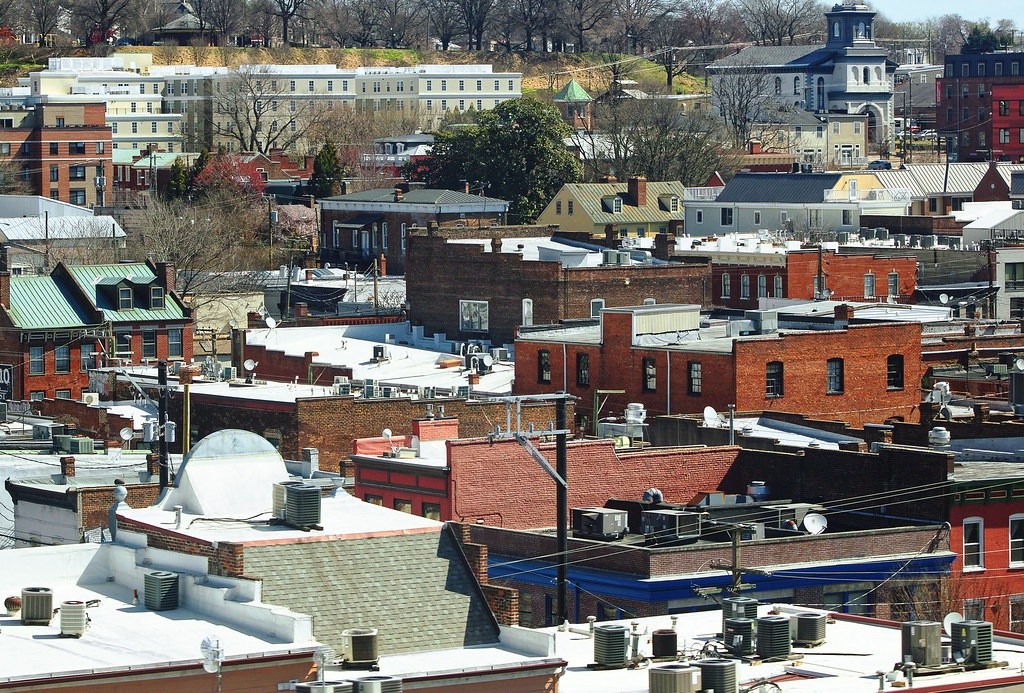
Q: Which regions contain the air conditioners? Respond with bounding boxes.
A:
[95,177,107,186]
[82,393,99,405]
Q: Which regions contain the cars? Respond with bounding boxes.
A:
[866,160,892,170]
[913,129,937,141]
